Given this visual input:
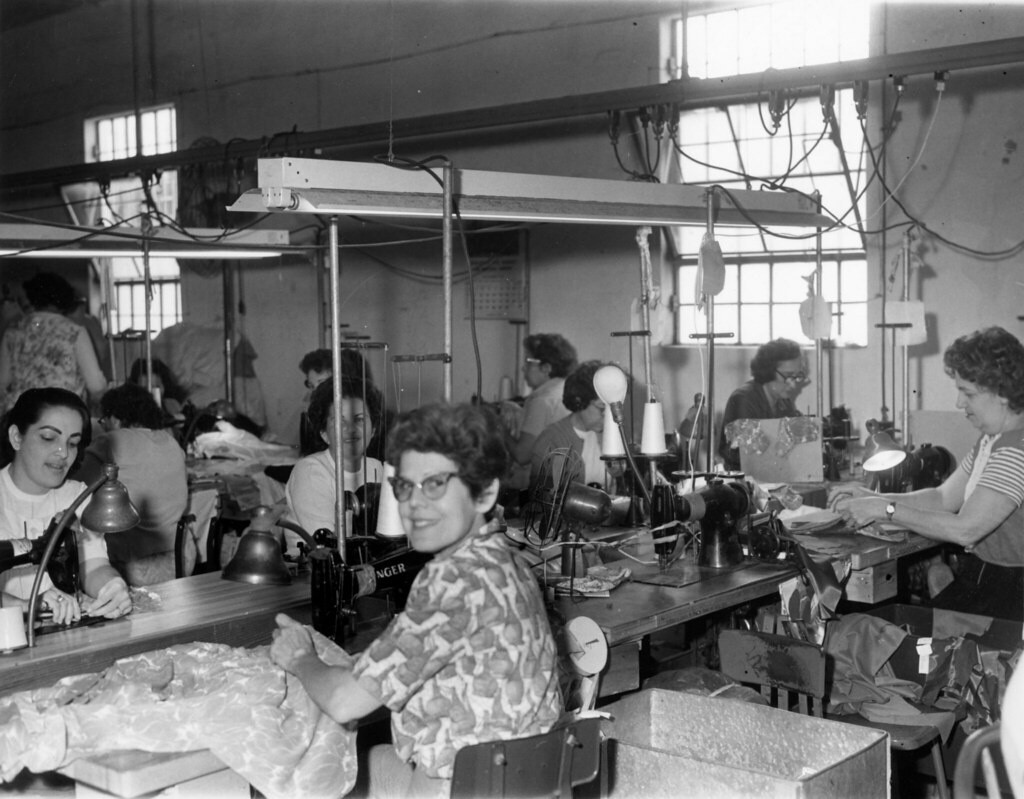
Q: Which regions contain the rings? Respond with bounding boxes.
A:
[117,607,123,615]
[57,596,64,602]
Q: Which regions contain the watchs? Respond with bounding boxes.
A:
[886,501,896,521]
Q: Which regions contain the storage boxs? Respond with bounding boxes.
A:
[594,687,893,799]
[857,605,1024,794]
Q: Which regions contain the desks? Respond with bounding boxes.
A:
[186,448,303,527]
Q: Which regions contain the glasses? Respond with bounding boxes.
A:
[592,403,604,415]
[524,356,542,366]
[387,472,459,503]
[775,370,805,385]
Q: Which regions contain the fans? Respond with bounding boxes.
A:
[526,447,614,599]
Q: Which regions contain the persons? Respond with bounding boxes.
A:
[827,325,1024,619]
[532,360,631,496]
[717,338,809,470]
[0,274,198,624]
[278,348,392,558]
[270,399,565,799]
[497,332,580,491]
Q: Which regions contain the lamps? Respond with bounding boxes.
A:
[30,461,142,649]
[221,504,318,587]
[861,418,908,495]
[225,158,839,228]
[680,394,706,494]
[183,398,236,452]
[592,365,653,504]
[0,220,290,259]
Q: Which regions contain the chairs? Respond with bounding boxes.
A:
[449,714,607,799]
[718,626,826,718]
